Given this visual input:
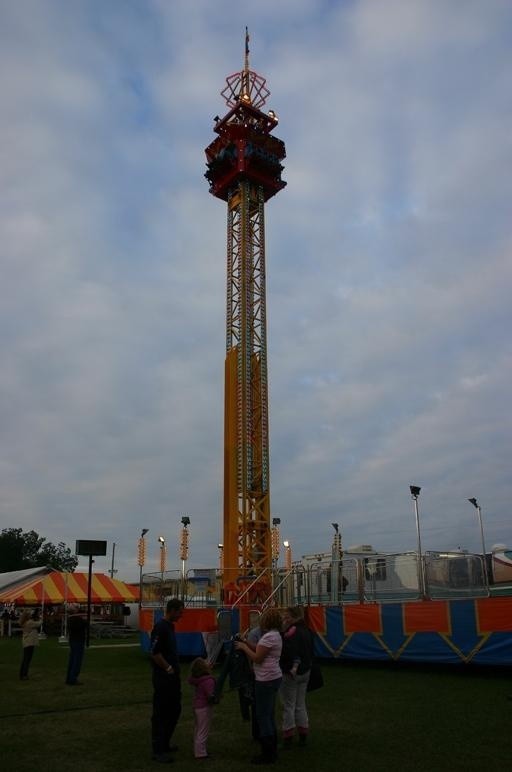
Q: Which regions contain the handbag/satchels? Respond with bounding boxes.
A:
[217,636,248,671]
[305,662,324,693]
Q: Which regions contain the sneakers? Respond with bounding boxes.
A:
[152,742,180,764]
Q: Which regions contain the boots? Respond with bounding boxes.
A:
[250,735,279,765]
[279,733,308,752]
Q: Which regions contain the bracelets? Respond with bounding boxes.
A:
[164,665,172,672]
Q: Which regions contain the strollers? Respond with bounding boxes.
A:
[204,629,261,722]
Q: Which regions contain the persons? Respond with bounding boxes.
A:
[0,609,10,636]
[188,657,220,764]
[149,598,181,765]
[18,608,44,680]
[66,610,88,686]
[9,610,19,638]
[343,576,346,591]
[30,608,42,634]
[235,606,316,765]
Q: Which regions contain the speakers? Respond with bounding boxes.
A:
[76,539,107,556]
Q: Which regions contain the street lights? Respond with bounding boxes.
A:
[328,519,344,592]
[135,515,295,613]
[408,484,431,599]
[468,496,488,597]
[74,534,109,649]
